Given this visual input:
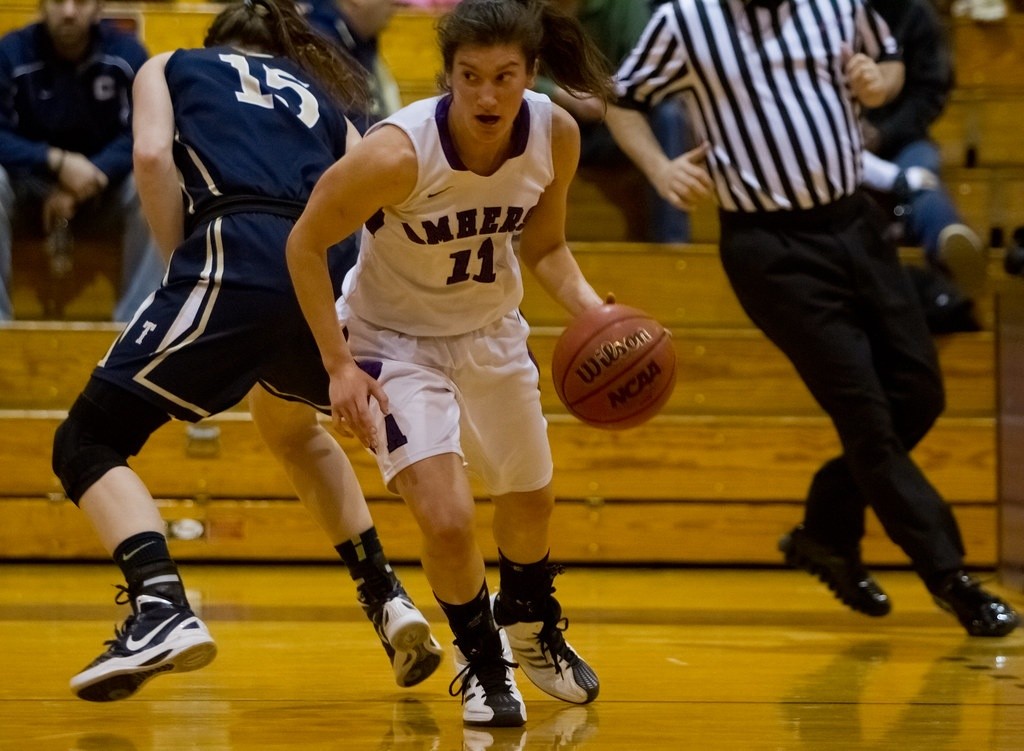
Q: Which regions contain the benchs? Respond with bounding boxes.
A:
[0,0,1023,562]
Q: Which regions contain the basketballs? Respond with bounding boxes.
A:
[549,302,679,432]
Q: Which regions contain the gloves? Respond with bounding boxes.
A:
[904,165,943,192]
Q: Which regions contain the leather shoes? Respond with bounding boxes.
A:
[780,523,893,616]
[934,571,1014,637]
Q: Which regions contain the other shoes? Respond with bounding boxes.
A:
[939,222,987,299]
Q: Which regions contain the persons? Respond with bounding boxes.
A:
[51,0,445,705]
[0,0,180,324]
[283,0,616,728]
[535,0,1024,637]
[287,0,416,118]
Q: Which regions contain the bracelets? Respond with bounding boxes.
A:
[51,147,67,173]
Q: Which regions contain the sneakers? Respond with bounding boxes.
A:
[490,584,601,704]
[452,625,527,726]
[357,578,445,687]
[68,581,218,703]
